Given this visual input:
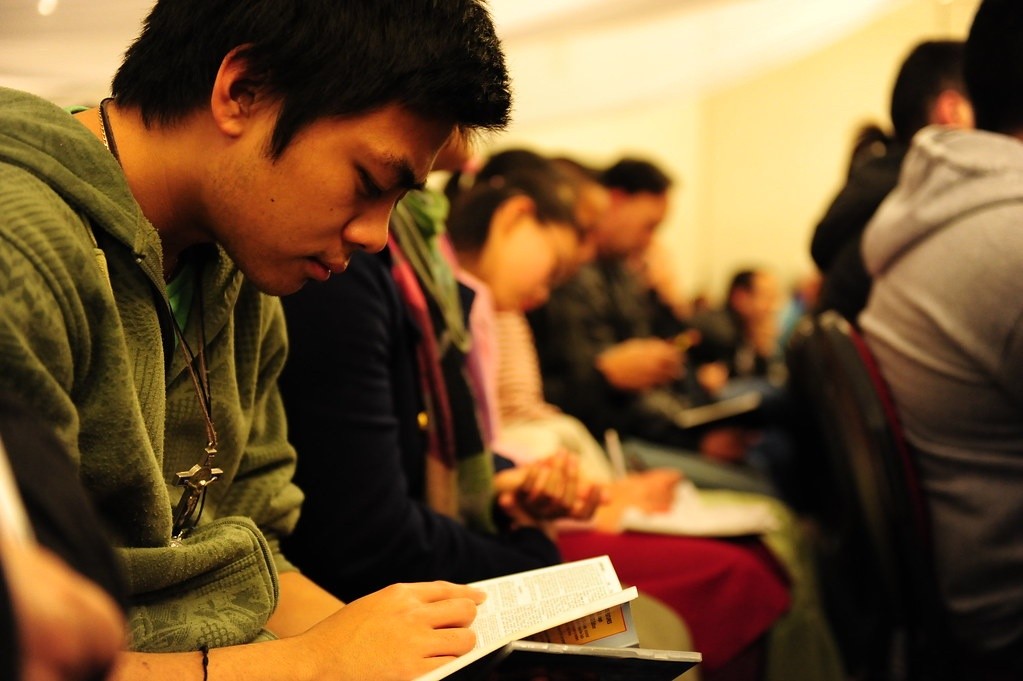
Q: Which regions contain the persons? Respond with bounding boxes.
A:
[0,0,511,681]
[446,149,795,592]
[277,129,792,681]
[859,0,1023,661]
[808,39,976,333]
[525,156,894,681]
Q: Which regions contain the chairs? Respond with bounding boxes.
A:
[777,325,912,681]
[817,306,1023,681]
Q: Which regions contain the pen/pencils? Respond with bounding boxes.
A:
[606,428,626,475]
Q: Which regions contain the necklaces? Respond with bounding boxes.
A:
[199,645,208,681]
[95,98,223,544]
